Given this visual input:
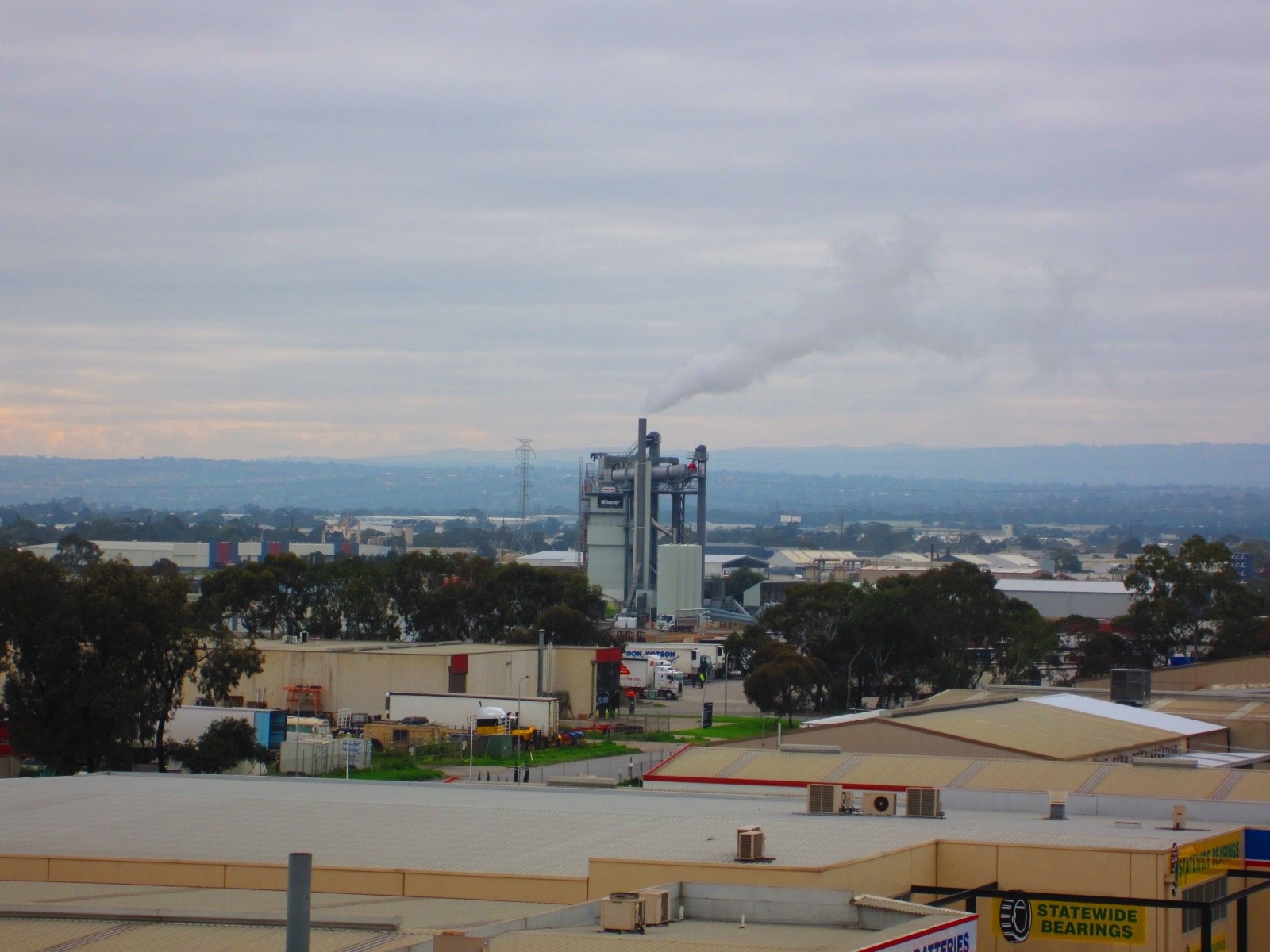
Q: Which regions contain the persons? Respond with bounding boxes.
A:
[691,670,705,689]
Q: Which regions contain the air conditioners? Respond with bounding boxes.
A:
[601,891,671,931]
[807,784,853,816]
[737,826,764,860]
[905,788,940,817]
[863,792,897,815]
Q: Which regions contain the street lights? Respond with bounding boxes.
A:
[725,651,731,715]
[517,675,534,763]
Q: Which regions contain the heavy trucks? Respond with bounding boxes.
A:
[625,645,713,681]
[656,608,709,633]
[619,653,683,700]
